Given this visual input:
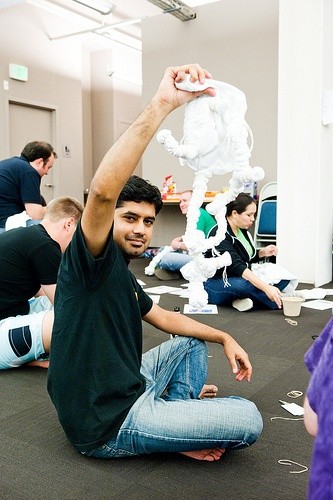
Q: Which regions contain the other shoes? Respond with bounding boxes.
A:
[154,268,180,281]
[281,291,306,304]
[232,298,254,312]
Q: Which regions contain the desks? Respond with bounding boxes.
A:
[161,199,212,205]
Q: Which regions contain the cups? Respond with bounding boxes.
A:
[280,296,305,317]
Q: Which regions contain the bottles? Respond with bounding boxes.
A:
[169,306,181,340]
[145,250,155,267]
[243,180,257,200]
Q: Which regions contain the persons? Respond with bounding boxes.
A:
[0,196,85,370]
[154,189,218,280]
[303,315,333,500]
[202,193,299,311]
[0,141,58,235]
[46,62,263,460]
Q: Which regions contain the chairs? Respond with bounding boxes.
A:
[253,182,277,264]
